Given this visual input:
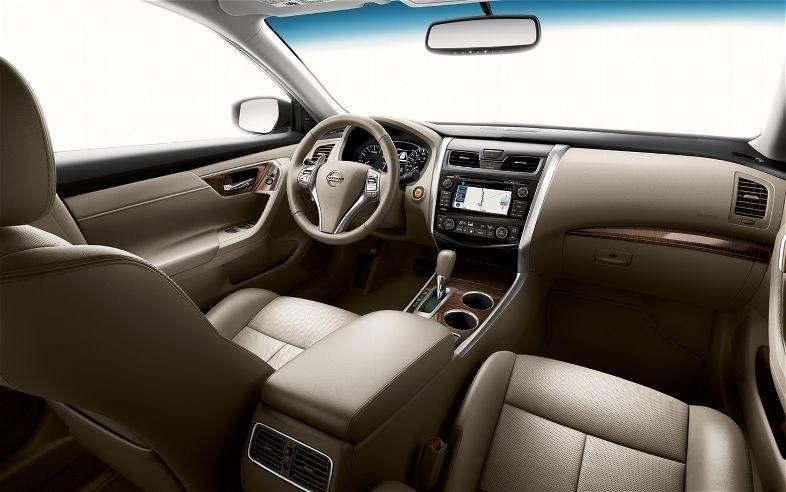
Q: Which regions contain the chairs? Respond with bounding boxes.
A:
[0,58,363,492]
[364,348,754,492]
